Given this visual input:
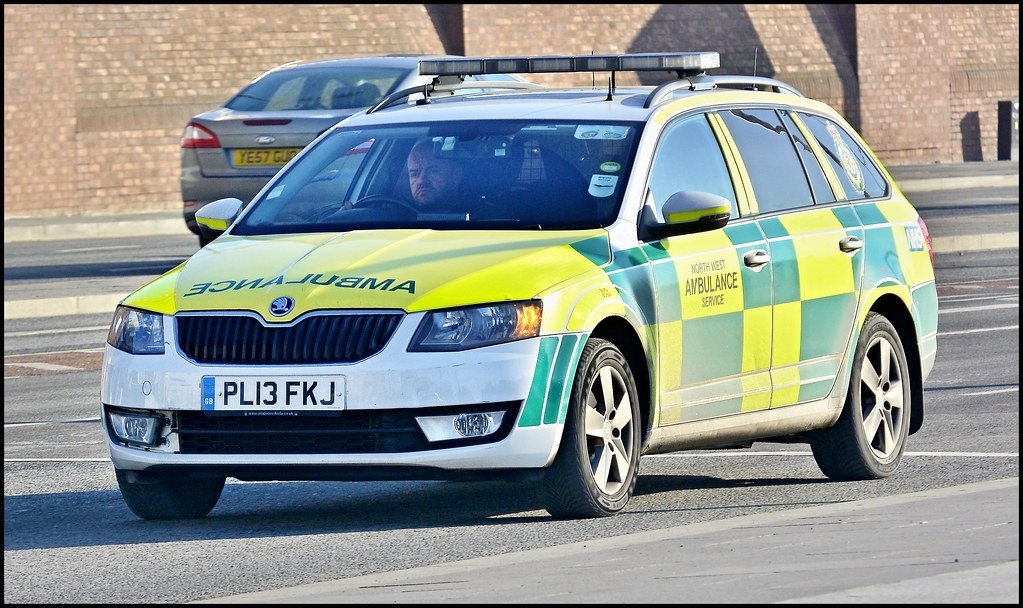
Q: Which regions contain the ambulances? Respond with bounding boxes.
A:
[95,54,939,523]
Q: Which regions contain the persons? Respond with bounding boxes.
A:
[339,136,507,220]
[353,83,381,107]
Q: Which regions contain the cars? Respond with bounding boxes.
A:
[179,55,529,252]
